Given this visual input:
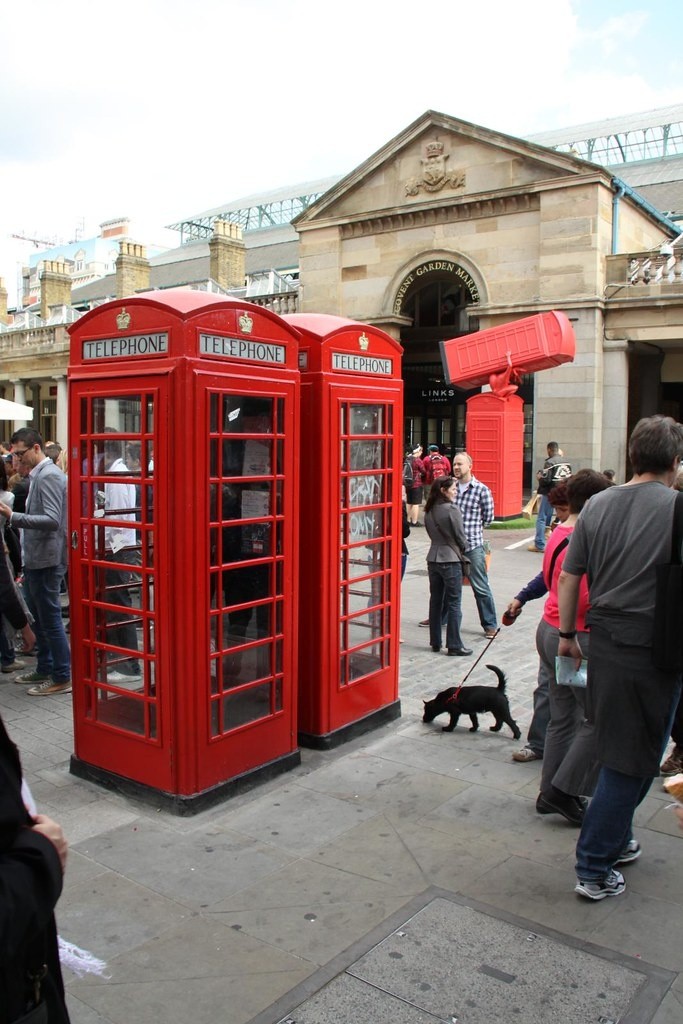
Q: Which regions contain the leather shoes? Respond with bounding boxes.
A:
[432,644,442,653]
[448,646,474,655]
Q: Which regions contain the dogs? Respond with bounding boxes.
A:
[422,665,522,742]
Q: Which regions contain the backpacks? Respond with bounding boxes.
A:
[403,456,420,487]
[429,454,449,483]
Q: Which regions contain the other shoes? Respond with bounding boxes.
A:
[486,625,498,640]
[536,783,589,824]
[660,744,683,775]
[13,641,38,654]
[2,659,26,673]
[418,619,431,626]
[106,669,141,684]
[60,604,72,619]
[512,747,539,763]
[529,544,546,554]
[545,520,558,540]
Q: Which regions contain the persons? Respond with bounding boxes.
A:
[365,479,411,644]
[403,444,426,527]
[527,440,572,553]
[0,720,72,1024]
[422,476,473,656]
[507,468,617,825]
[211,399,280,706]
[418,453,497,639]
[556,415,683,904]
[545,448,564,541]
[422,445,451,505]
[0,427,154,695]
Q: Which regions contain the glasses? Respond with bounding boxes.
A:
[13,443,35,458]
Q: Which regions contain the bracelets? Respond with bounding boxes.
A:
[559,630,578,639]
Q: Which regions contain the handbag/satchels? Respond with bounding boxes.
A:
[462,544,491,587]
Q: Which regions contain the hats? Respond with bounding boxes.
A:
[428,444,440,452]
[410,443,422,455]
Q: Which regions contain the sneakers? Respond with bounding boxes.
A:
[574,871,628,901]
[27,677,74,696]
[15,670,52,684]
[612,840,643,867]
